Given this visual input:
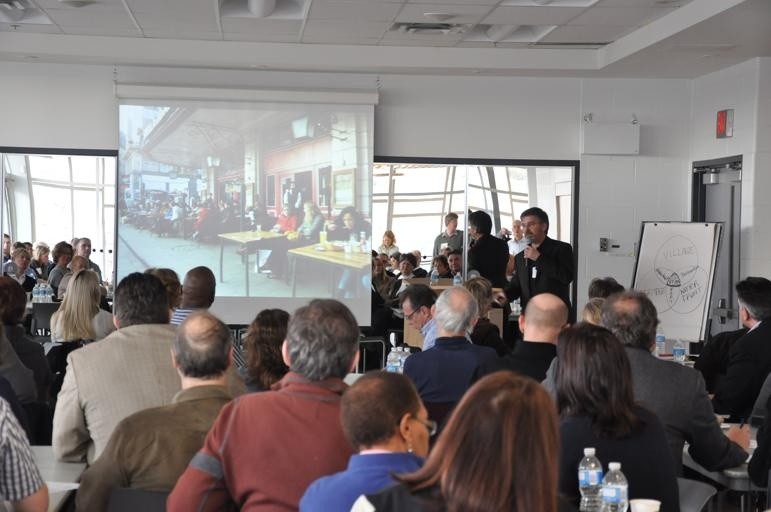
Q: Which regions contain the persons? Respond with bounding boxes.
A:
[284,185,291,206]
[539,289,753,512]
[372,250,388,271]
[426,255,453,278]
[504,220,532,281]
[358,258,400,347]
[50,272,116,347]
[4,248,37,291]
[499,293,569,383]
[296,186,306,206]
[377,231,399,254]
[397,283,436,352]
[298,370,436,511]
[237,309,289,395]
[57,256,89,300]
[408,250,428,278]
[461,278,504,353]
[166,297,358,512]
[350,369,582,511]
[74,237,102,286]
[0,277,51,446]
[433,213,467,260]
[0,396,50,512]
[402,287,503,455]
[51,272,252,467]
[326,205,372,298]
[588,276,625,300]
[395,253,423,280]
[75,309,238,512]
[439,250,492,300]
[501,207,577,324]
[47,241,71,290]
[695,328,753,391]
[259,200,325,278]
[23,242,34,258]
[119,192,275,242]
[467,210,509,286]
[289,181,296,205]
[4,234,11,264]
[710,276,771,424]
[492,228,512,241]
[271,202,306,234]
[143,267,182,309]
[30,242,55,281]
[171,266,247,371]
[385,251,403,280]
[556,323,680,511]
[579,296,604,327]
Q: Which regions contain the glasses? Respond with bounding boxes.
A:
[415,417,437,437]
[404,303,427,320]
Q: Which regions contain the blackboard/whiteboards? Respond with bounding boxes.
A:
[631,221,724,345]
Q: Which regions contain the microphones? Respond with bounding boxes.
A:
[526,234,536,270]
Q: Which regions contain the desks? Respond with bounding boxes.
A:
[5,445,87,512]
[217,230,288,297]
[681,428,768,512]
[287,242,371,300]
[343,372,365,386]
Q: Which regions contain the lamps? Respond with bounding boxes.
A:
[0,0,24,23]
[485,19,522,41]
[246,0,276,19]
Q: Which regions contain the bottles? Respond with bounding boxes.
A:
[46,285,53,302]
[348,229,359,256]
[454,272,463,286]
[580,448,602,511]
[360,232,369,257]
[430,267,440,287]
[397,346,403,360]
[601,462,628,512]
[387,348,400,373]
[39,283,46,303]
[401,348,411,375]
[32,285,39,304]
[655,339,665,360]
[673,338,685,366]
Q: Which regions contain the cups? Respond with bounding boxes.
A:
[514,300,520,316]
[630,498,661,512]
